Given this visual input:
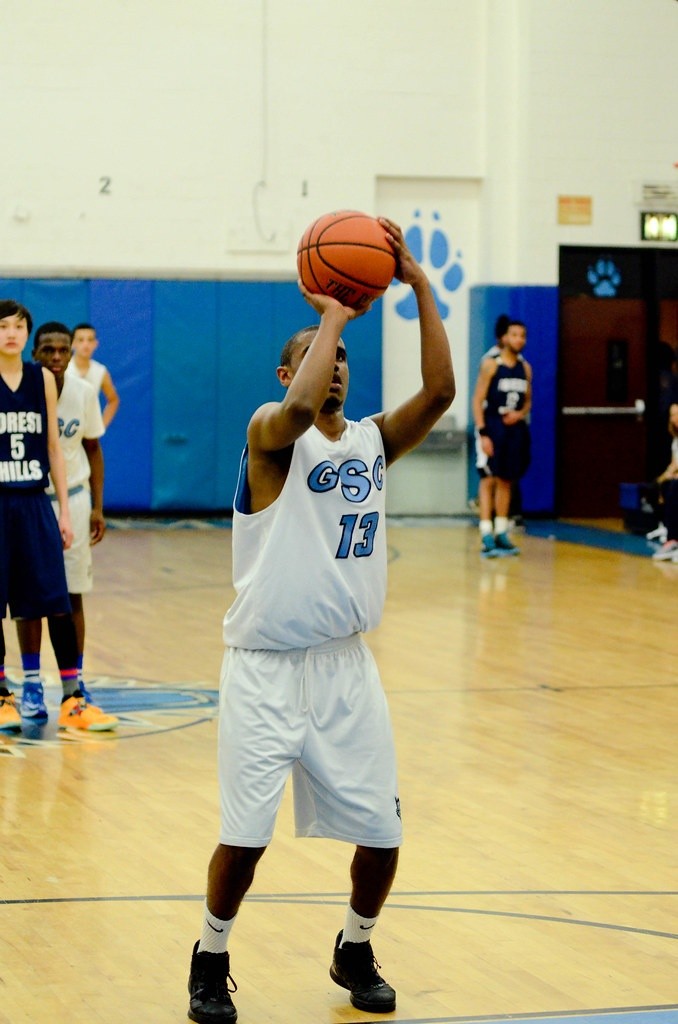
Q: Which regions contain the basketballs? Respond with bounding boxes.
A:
[297,208,396,308]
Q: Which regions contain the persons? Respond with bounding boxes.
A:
[470,315,678,562]
[187,216,456,1024]
[0,300,120,732]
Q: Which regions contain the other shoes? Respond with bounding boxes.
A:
[649,538,678,561]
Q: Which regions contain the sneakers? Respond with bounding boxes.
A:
[0,686,22,731]
[479,531,519,561]
[56,689,121,732]
[79,681,94,704]
[329,929,396,1013]
[17,682,48,723]
[186,939,240,1024]
